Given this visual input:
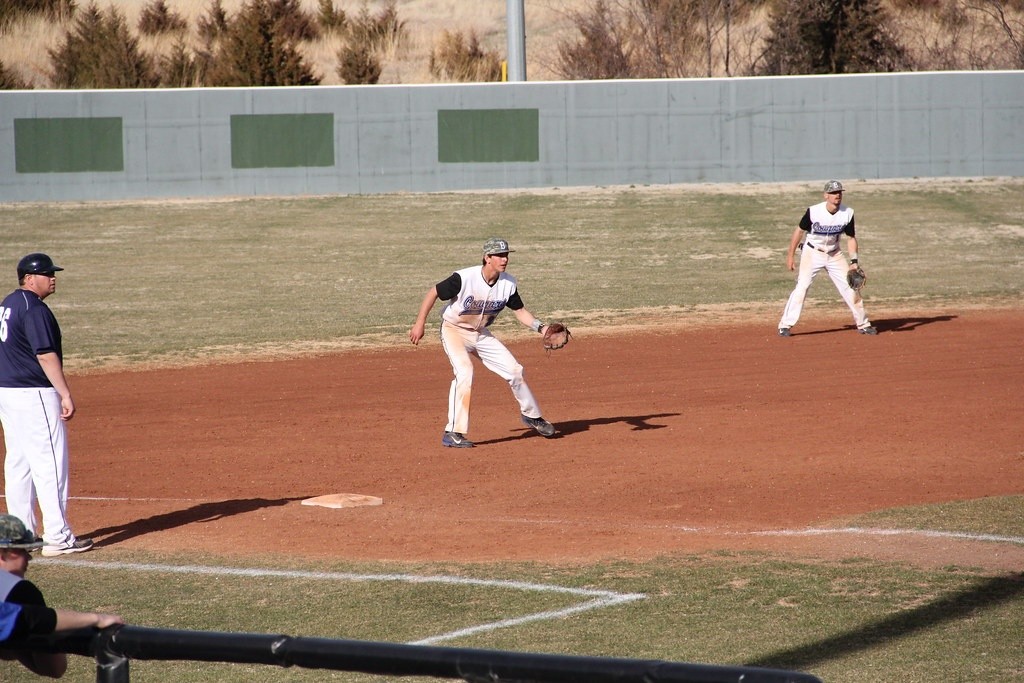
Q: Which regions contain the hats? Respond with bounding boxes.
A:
[824,181,845,192]
[0,515,48,550]
[483,238,515,255]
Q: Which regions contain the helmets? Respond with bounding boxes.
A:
[17,254,63,280]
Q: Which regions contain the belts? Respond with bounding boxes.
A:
[807,242,835,253]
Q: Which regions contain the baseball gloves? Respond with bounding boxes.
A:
[846,265,867,291]
[542,323,574,359]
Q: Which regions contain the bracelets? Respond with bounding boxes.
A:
[850,253,858,259]
[850,259,857,265]
[538,324,545,333]
[531,319,542,331]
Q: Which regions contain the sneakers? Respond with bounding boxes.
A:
[777,327,790,337]
[40,539,94,556]
[520,414,555,437]
[858,327,878,335]
[442,432,473,447]
[31,537,43,552]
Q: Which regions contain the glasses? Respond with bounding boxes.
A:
[0,529,35,545]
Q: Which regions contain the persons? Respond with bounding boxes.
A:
[0,514,124,678]
[0,254,93,556]
[777,180,878,336]
[412,236,572,448]
